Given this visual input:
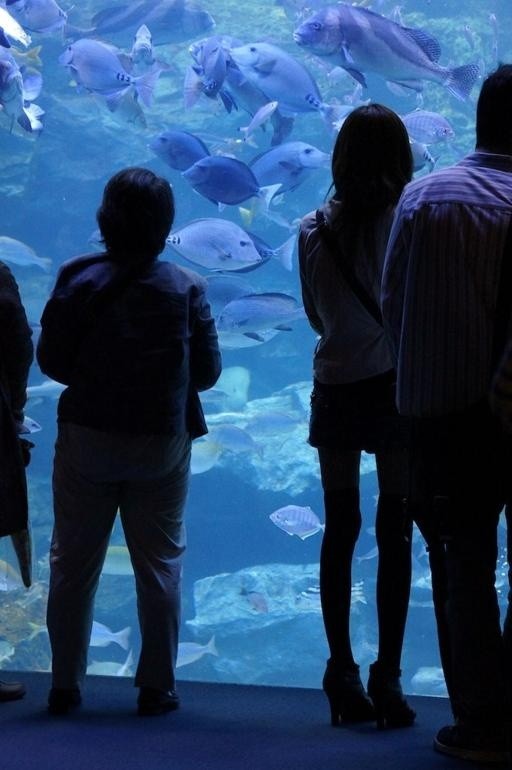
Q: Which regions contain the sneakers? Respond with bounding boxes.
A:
[433,725,511,762]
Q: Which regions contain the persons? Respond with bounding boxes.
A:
[298,101,415,734]
[35,160,221,721]
[0,260,35,704]
[380,57,511,757]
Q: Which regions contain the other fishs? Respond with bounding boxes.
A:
[0,0,510,700]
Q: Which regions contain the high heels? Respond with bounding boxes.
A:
[367,664,416,730]
[323,658,374,726]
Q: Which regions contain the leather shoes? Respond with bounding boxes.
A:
[136,688,179,715]
[0,682,27,701]
